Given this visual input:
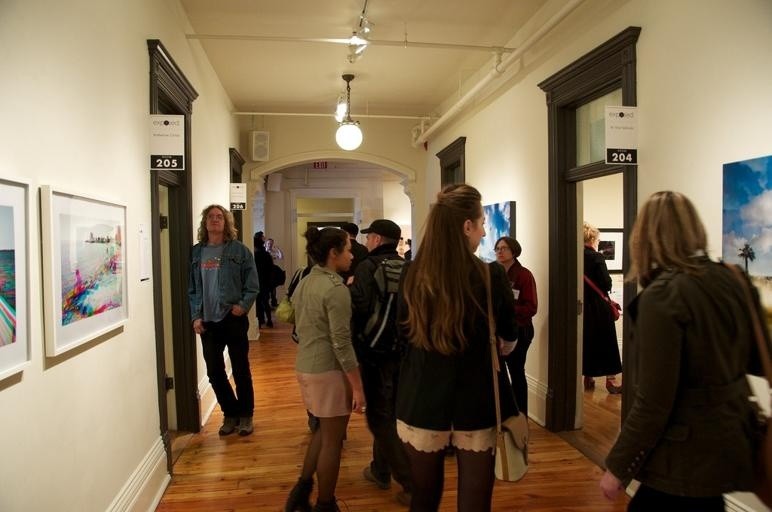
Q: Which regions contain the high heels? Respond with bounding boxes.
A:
[584,379,595,389]
[605,380,624,394]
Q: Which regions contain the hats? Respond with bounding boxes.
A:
[361,220,404,241]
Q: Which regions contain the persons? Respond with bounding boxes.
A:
[347,219,408,490]
[597,189,772,512]
[395,181,520,512]
[284,228,366,512]
[582,221,622,396]
[493,236,538,424]
[339,223,369,288]
[254,232,274,329]
[265,238,283,307]
[187,204,258,435]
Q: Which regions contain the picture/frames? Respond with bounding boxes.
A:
[474,201,516,265]
[41,184,132,357]
[594,228,623,273]
[0,177,32,381]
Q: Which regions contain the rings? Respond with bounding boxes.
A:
[361,407,365,413]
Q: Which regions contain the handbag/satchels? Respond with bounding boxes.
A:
[583,273,621,322]
[275,266,306,323]
[484,262,530,483]
[723,262,771,511]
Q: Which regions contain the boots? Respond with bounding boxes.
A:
[285,478,312,512]
[314,497,349,511]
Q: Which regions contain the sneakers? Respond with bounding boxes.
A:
[364,466,390,489]
[239,416,254,434]
[219,414,239,436]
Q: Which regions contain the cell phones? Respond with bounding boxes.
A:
[352,400,366,413]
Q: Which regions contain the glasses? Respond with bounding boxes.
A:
[493,246,510,252]
[205,214,224,220]
[598,239,601,241]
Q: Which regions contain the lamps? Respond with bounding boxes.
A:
[336,73,363,151]
